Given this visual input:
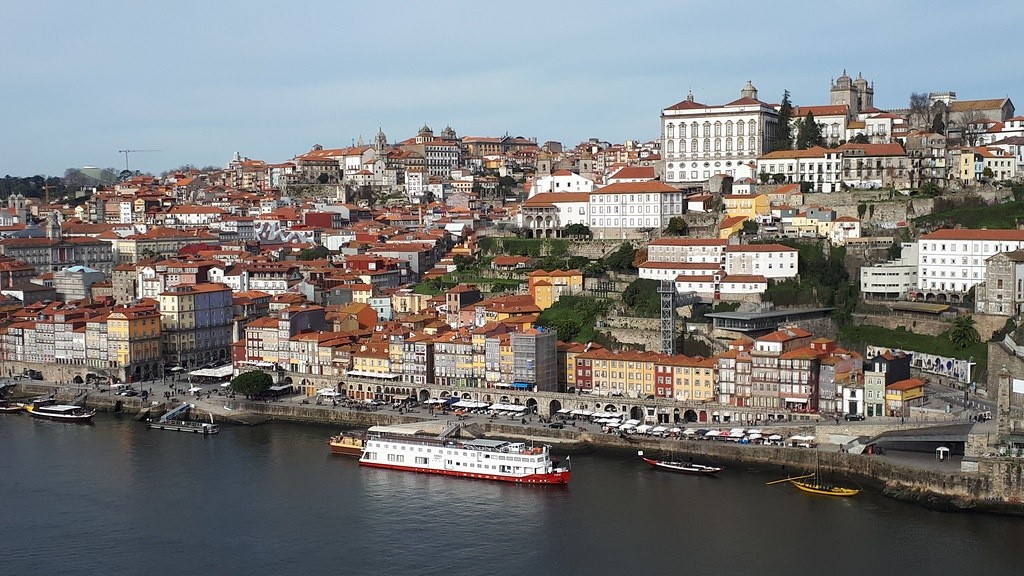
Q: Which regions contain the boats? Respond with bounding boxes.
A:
[326,420,572,485]
[0,380,97,422]
[146,418,219,434]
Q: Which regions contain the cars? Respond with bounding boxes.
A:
[115,389,148,397]
[548,422,563,429]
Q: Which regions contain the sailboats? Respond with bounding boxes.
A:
[640,423,724,475]
[790,444,864,495]
